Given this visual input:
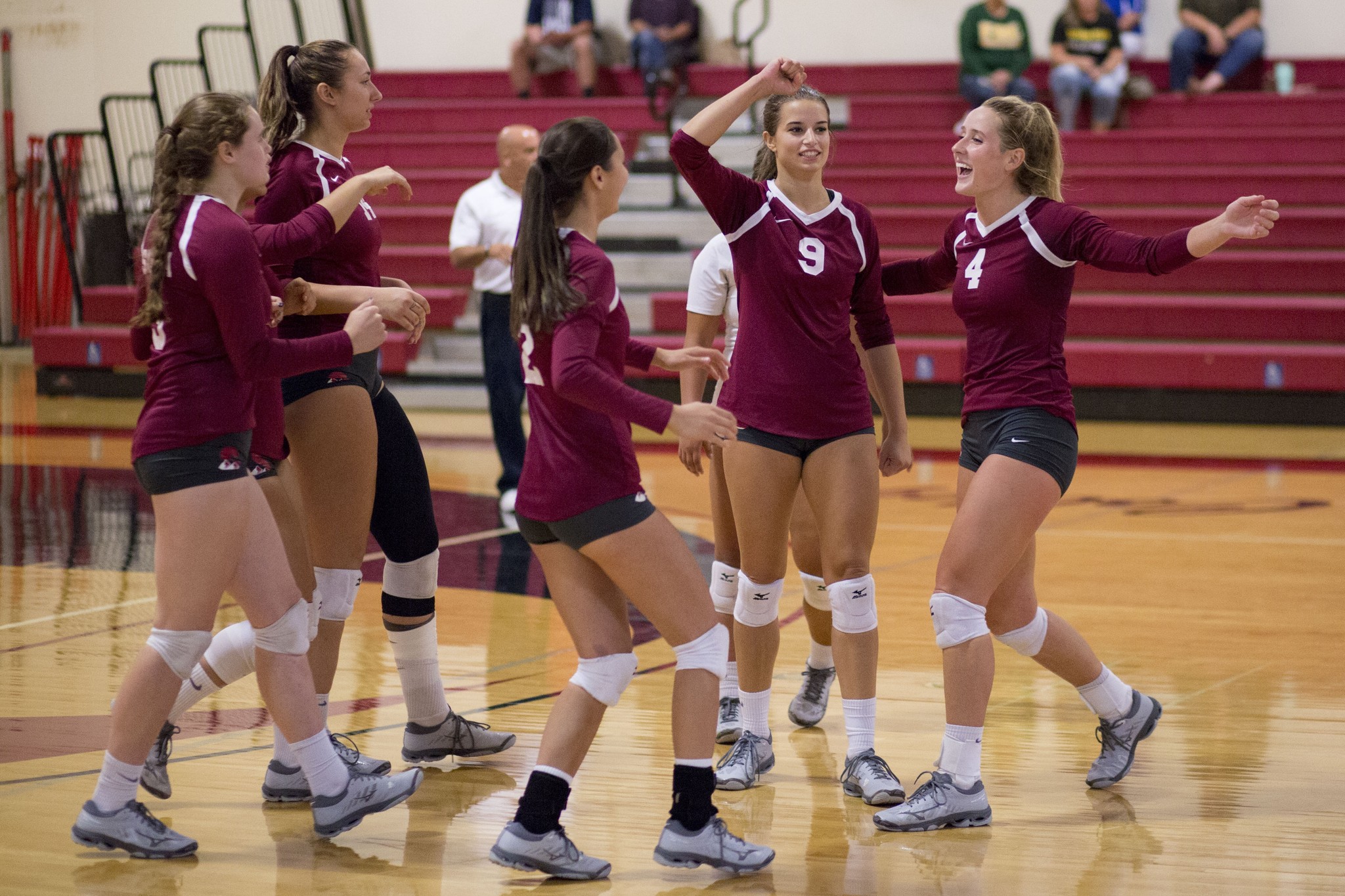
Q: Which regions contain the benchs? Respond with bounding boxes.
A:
[29,50,1345,429]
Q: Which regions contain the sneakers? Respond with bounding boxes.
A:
[789,727,840,782]
[403,767,517,815]
[401,703,516,763]
[1085,689,1163,789]
[73,856,200,892]
[504,875,613,894]
[276,840,406,895]
[654,873,778,894]
[1090,789,1163,858]
[264,802,316,839]
[787,656,836,727]
[71,799,198,859]
[840,749,907,806]
[875,833,991,867]
[328,732,392,775]
[872,770,993,832]
[261,759,313,802]
[842,795,892,847]
[712,787,775,827]
[487,821,612,879]
[713,728,776,790]
[310,767,424,839]
[139,720,181,800]
[715,697,744,743]
[651,813,775,876]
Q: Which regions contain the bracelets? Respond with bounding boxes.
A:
[483,244,492,258]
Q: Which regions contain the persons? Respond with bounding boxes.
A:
[1169,0,1265,97]
[1103,0,1148,58]
[448,120,543,513]
[677,147,892,742]
[953,0,1037,134]
[486,117,775,881]
[513,0,601,102]
[626,0,702,98]
[69,96,425,864]
[139,41,518,801]
[1048,0,1128,133]
[872,95,1279,834]
[668,59,914,806]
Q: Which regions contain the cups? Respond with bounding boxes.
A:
[1275,62,1292,93]
